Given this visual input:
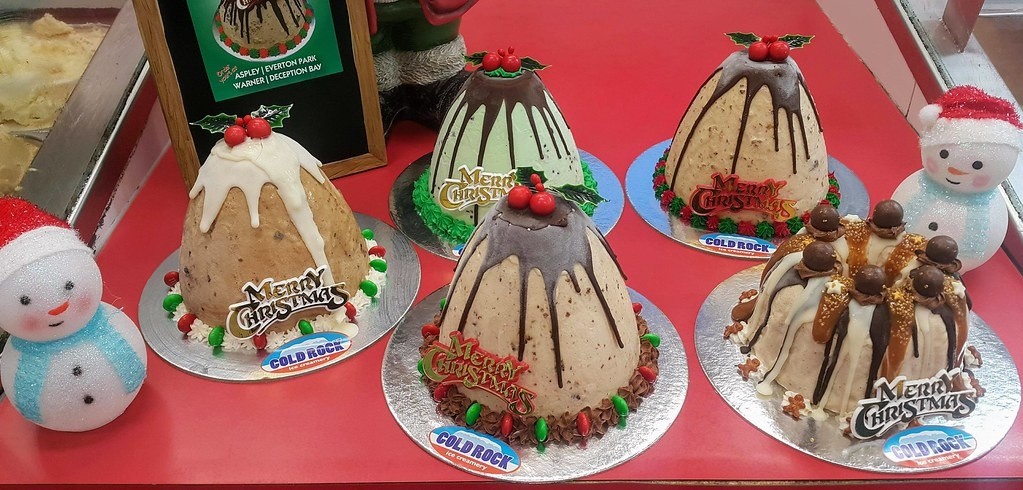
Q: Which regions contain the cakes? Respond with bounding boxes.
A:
[161,104,386,349]
[413,34,985,453]
